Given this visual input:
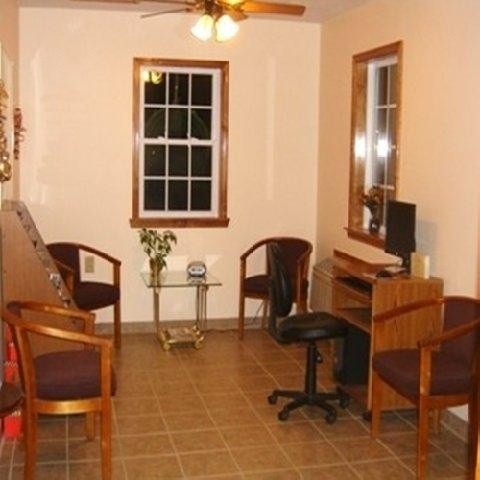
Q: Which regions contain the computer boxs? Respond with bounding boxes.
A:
[332,324,371,385]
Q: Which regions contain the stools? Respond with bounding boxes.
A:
[268,311,349,425]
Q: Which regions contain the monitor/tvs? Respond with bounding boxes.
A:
[384,198,417,274]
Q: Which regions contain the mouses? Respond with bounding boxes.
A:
[376,270,393,277]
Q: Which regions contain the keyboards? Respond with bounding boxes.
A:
[337,276,372,293]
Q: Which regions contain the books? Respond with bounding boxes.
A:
[410,252,430,280]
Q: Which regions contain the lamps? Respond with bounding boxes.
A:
[185,1,247,42]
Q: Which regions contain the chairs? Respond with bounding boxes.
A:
[237,236,313,342]
[370,296,478,479]
[0,241,123,480]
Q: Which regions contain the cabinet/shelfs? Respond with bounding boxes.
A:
[140,272,222,350]
[309,248,442,421]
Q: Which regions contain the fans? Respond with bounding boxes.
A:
[134,0,305,23]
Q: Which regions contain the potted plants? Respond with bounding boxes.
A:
[359,188,385,235]
[137,227,176,280]
